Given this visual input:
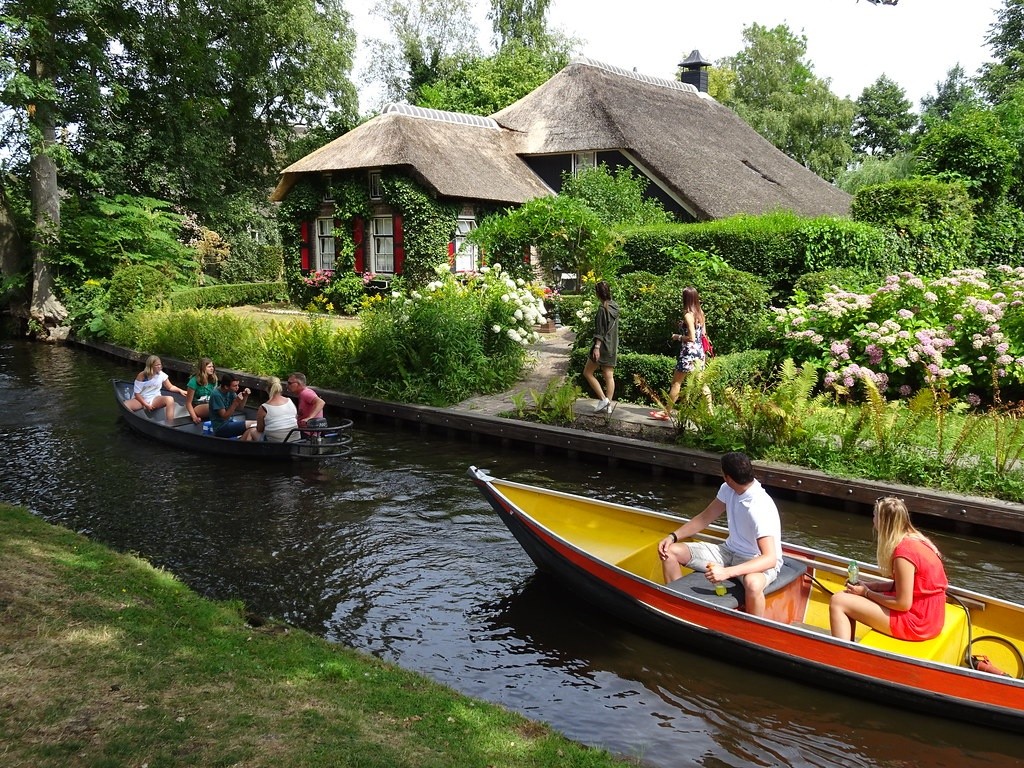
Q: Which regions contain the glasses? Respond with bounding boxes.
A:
[288,381,298,385]
[875,496,883,505]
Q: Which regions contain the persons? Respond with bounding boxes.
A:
[240,376,301,443]
[650,286,713,423]
[658,452,784,618]
[209,372,257,438]
[187,358,219,424]
[125,355,187,426]
[584,280,621,414]
[287,372,326,440]
[829,497,948,642]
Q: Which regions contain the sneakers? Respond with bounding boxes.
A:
[592,398,611,413]
[599,404,611,413]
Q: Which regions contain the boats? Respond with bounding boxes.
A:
[108,375,356,459]
[466,462,1024,731]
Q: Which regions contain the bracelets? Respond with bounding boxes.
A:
[594,345,600,348]
[669,532,677,543]
[864,589,870,598]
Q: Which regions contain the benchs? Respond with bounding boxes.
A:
[157,409,245,434]
[858,600,969,671]
[667,556,807,625]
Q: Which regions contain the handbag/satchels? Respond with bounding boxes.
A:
[702,333,712,357]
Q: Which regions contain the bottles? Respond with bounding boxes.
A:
[848,561,859,585]
[708,562,728,596]
[124,385,131,399]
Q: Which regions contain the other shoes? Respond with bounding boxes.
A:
[649,411,669,421]
